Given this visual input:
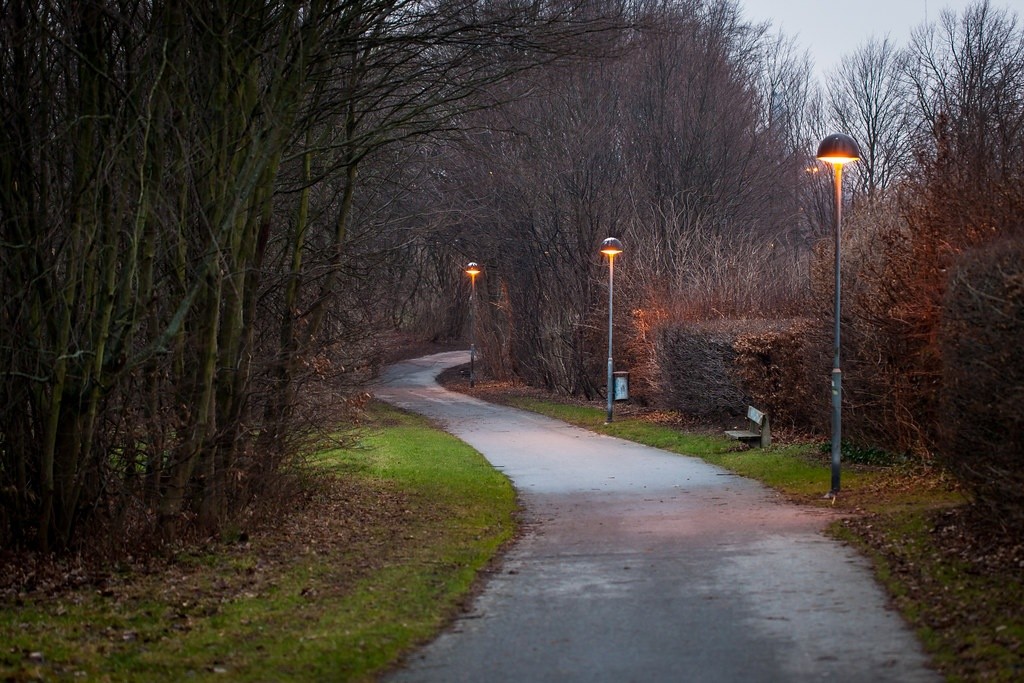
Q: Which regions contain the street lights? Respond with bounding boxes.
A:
[817,129,862,491]
[599,237,624,425]
[465,263,480,387]
